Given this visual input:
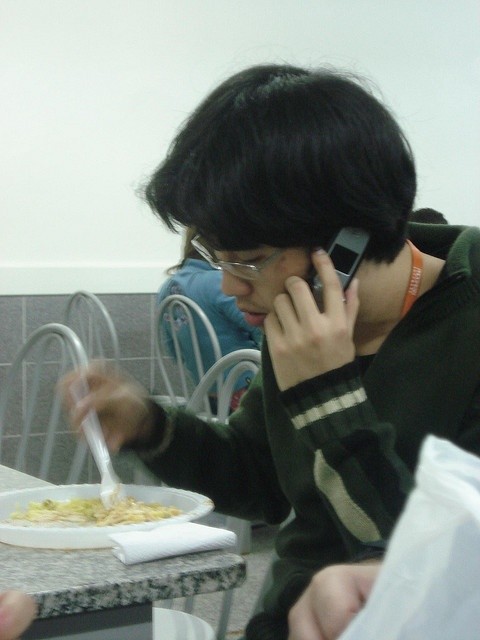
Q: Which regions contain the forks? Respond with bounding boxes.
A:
[65,371,127,507]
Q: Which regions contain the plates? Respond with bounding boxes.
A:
[1,483,214,550]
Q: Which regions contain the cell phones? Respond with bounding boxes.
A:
[310,226,374,313]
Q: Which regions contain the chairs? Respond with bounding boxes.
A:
[156,294,224,426]
[0,322,91,487]
[183,348,263,429]
[64,291,121,404]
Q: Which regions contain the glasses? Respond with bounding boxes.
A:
[191,233,283,281]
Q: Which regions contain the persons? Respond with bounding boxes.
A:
[2,592,36,640]
[285,557,384,640]
[54,64,480,640]
[157,223,266,418]
